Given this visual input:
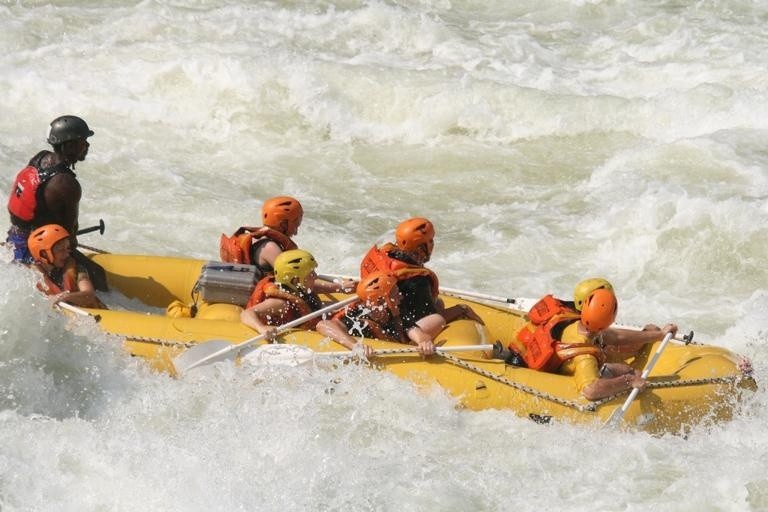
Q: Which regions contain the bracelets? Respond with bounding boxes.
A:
[622,374,632,390]
[455,302,469,315]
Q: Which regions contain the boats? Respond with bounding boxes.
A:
[52,250,758,439]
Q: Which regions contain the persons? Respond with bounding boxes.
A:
[360,215,484,342]
[510,277,679,365]
[520,292,648,401]
[5,114,111,295]
[27,222,107,309]
[217,194,354,301]
[236,247,323,343]
[315,271,436,362]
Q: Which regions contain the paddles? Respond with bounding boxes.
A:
[508,296,693,345]
[257,341,504,368]
[172,296,361,382]
[595,331,673,442]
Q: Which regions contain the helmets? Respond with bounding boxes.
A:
[574,277,614,313]
[26,223,71,267]
[47,114,95,145]
[579,288,618,334]
[355,269,398,302]
[273,249,320,293]
[261,196,304,237]
[394,215,435,252]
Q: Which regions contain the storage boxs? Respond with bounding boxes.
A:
[201,260,261,306]
